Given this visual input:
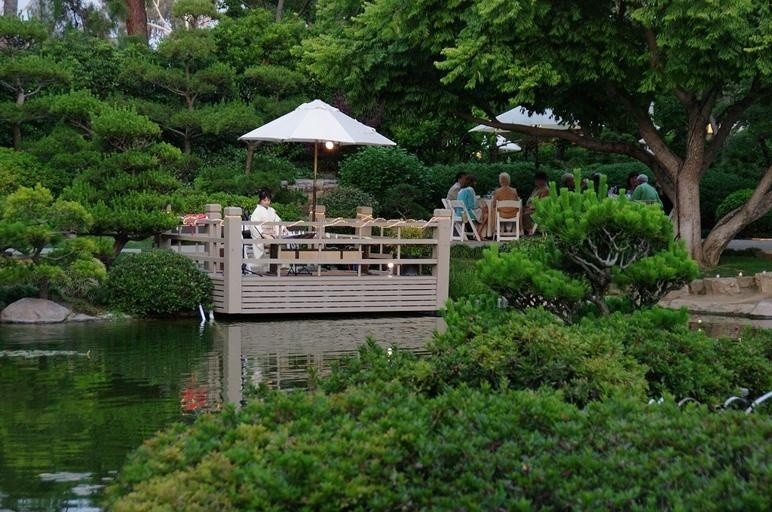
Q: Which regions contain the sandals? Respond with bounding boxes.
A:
[474,233,487,241]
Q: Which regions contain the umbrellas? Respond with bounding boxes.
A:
[237,99,397,249]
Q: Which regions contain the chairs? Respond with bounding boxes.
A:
[442,198,522,244]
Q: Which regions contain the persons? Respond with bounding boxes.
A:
[523,171,549,236]
[447,172,488,241]
[492,172,519,232]
[558,171,663,209]
[249,190,289,259]
